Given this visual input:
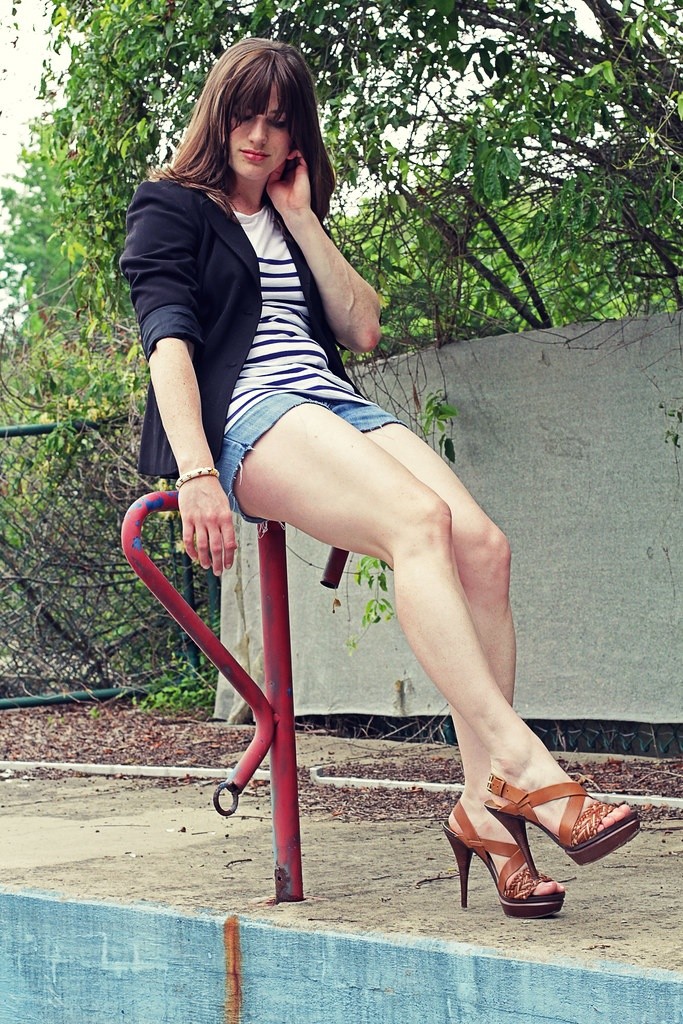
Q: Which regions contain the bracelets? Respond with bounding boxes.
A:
[175,467,220,491]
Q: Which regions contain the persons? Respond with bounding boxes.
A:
[118,37,641,919]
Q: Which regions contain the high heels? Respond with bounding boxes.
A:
[484,774,640,876]
[441,799,566,919]
